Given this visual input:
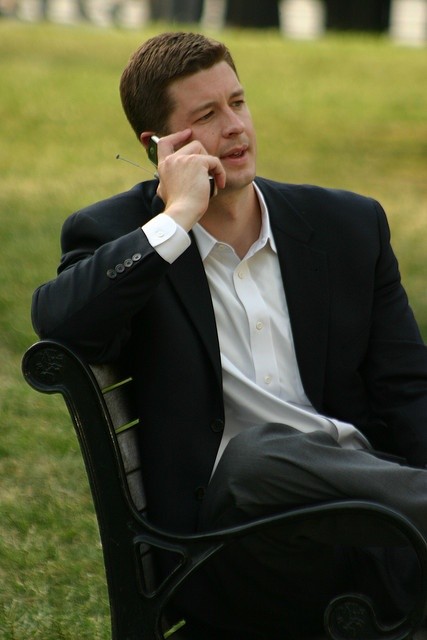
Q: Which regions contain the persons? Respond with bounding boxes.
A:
[30,32,427,533]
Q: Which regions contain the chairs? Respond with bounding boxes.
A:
[22,339,426,638]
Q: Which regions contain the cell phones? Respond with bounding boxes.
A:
[146,135,219,197]
